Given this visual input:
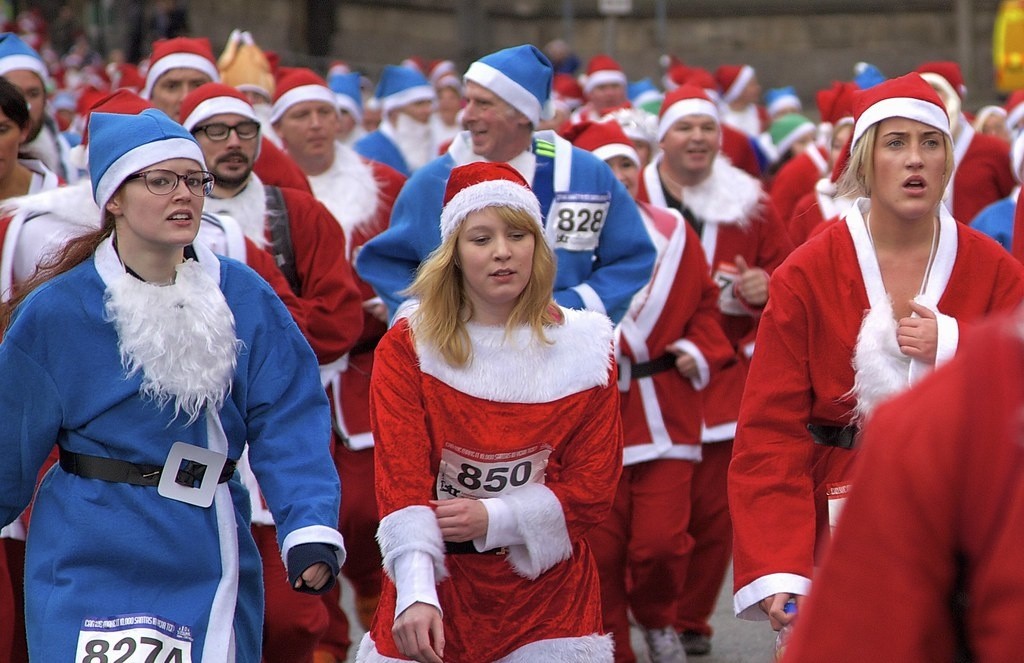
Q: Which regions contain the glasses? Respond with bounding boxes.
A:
[191,122,260,140]
[124,169,217,198]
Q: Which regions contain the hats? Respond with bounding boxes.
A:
[71,91,156,170]
[914,63,1024,182]
[440,162,546,245]
[180,82,259,133]
[550,54,887,168]
[464,44,556,129]
[0,10,461,124]
[89,108,210,213]
[815,71,954,196]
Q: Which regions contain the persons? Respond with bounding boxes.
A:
[777,325,1023,662]
[0,28,1024,663]
[728,73,1024,644]
[350,161,622,663]
[0,108,347,662]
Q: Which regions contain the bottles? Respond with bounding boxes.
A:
[774,603,797,658]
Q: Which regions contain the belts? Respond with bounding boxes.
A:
[614,353,677,392]
[349,338,380,356]
[443,540,510,556]
[720,344,739,371]
[59,441,237,508]
[807,423,859,449]
